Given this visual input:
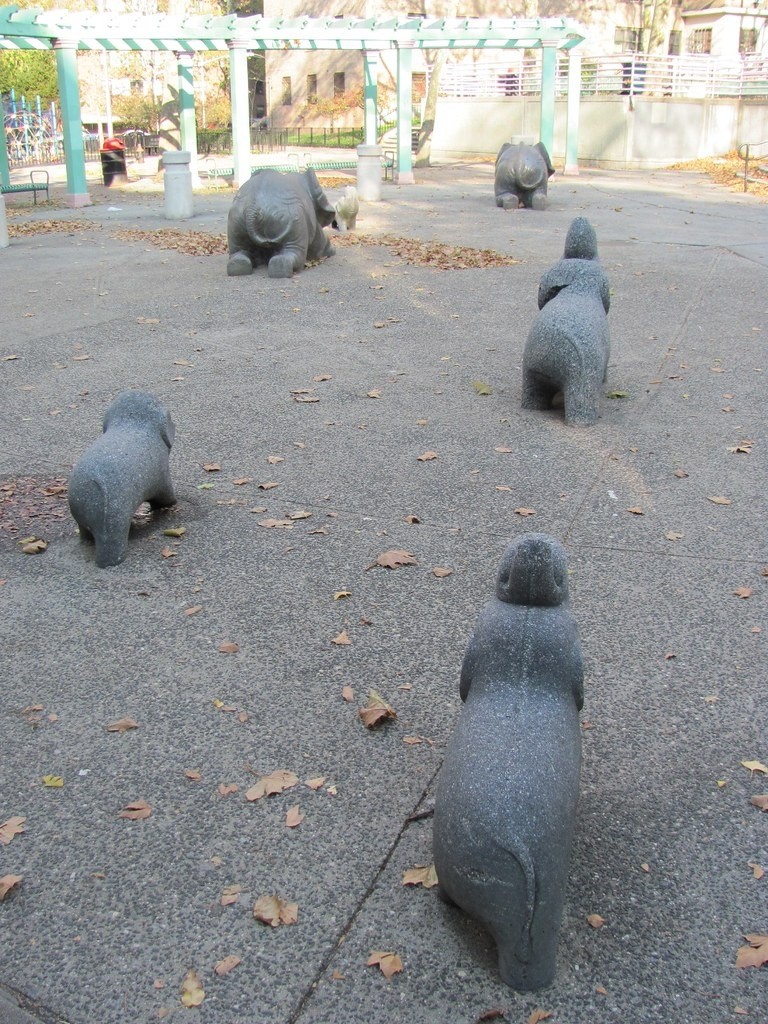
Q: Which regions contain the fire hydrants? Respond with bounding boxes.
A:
[135,143,145,164]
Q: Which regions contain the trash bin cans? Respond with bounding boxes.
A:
[100,149,128,187]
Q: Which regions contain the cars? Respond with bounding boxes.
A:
[121,129,152,137]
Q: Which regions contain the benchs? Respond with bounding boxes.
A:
[304,151,395,182]
[205,153,300,192]
[0,170,50,206]
